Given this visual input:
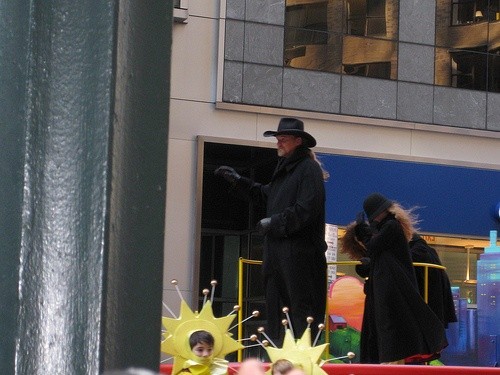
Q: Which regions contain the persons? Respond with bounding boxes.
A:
[212,117,329,344]
[335,217,458,330]
[158,330,304,375]
[354,193,456,365]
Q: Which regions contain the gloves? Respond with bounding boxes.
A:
[215,166,241,184]
[360,257,371,265]
[357,211,364,225]
[252,217,272,241]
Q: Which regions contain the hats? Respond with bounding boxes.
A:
[363,193,393,221]
[263,117,316,148]
